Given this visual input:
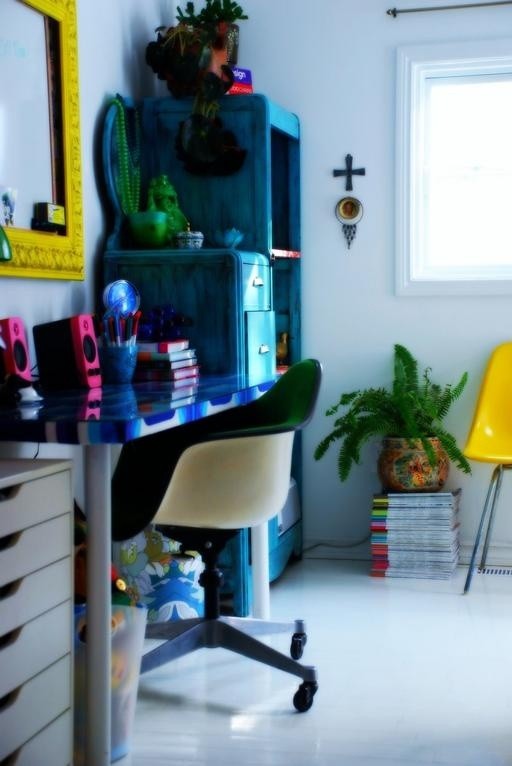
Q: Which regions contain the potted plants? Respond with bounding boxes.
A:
[312,342,473,493]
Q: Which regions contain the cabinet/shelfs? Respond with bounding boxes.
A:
[0,456,77,765]
[101,248,276,391]
[141,91,302,616]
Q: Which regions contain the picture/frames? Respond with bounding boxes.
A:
[0,0,89,281]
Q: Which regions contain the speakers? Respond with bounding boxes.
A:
[0,317,32,387]
[77,389,103,421]
[32,314,103,389]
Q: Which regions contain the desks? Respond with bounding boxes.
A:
[1,376,268,765]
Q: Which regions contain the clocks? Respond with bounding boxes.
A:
[102,278,141,320]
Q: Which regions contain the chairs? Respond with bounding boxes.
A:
[110,359,323,710]
[463,341,512,592]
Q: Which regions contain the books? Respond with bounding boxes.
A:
[271,248,301,259]
[135,340,201,381]
[134,376,199,411]
[368,490,461,579]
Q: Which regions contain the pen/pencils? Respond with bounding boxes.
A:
[98,311,142,348]
